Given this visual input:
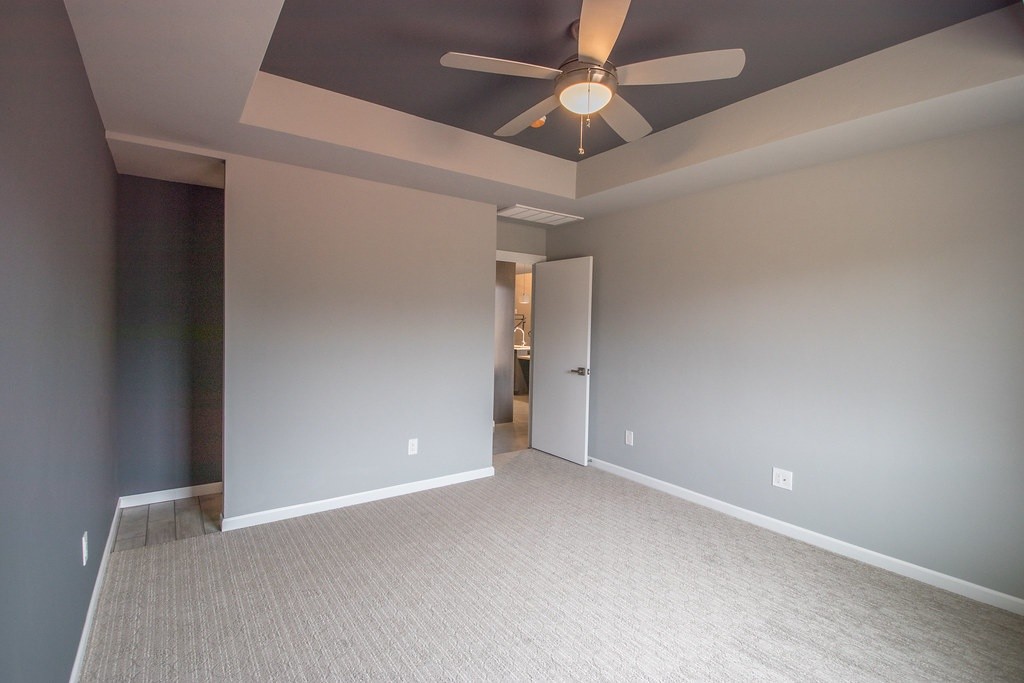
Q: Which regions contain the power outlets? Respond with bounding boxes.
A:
[626,430,634,446]
[409,438,418,455]
[772,468,793,490]
[82,531,89,565]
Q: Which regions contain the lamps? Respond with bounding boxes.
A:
[554,62,617,155]
[519,264,530,303]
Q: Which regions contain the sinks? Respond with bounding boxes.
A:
[514,346,530,395]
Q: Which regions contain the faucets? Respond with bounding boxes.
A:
[514,327,526,347]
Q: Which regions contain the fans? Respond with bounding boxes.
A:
[440,0,746,143]
